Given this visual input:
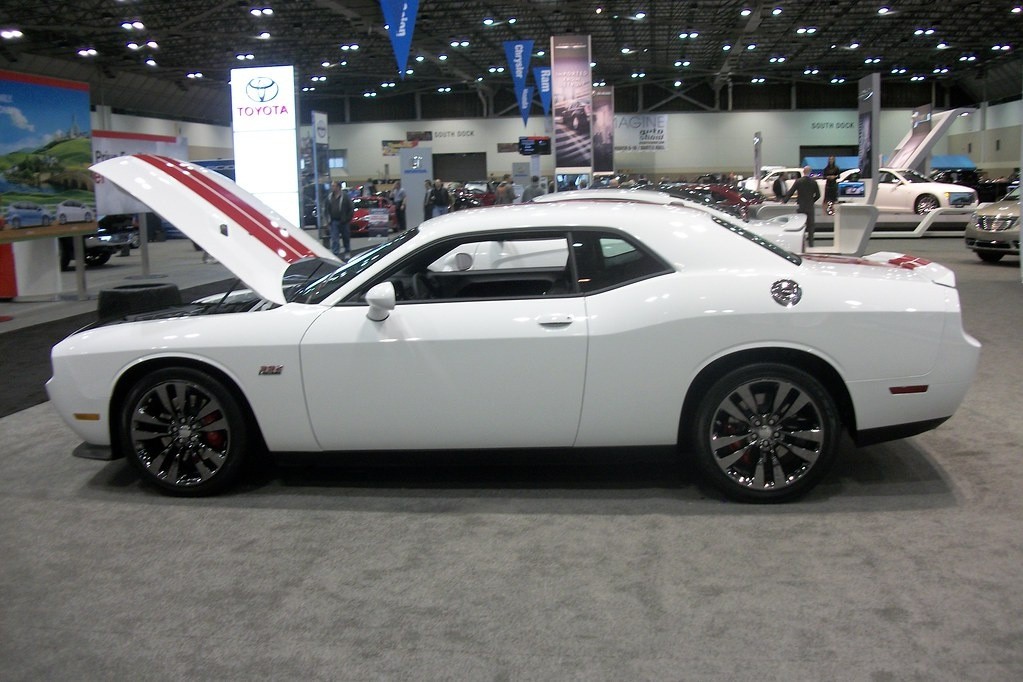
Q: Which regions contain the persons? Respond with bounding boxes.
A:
[773,172,787,204]
[780,166,821,248]
[1007,167,1021,185]
[823,154,840,216]
[363,173,688,234]
[324,180,354,256]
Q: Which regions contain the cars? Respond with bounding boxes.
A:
[561,99,590,131]
[7,201,56,230]
[55,199,94,228]
[442,180,524,209]
[301,182,331,226]
[963,185,1020,264]
[631,165,1019,216]
[59,227,142,272]
[347,197,398,236]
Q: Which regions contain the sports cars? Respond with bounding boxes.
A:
[43,152,985,499]
